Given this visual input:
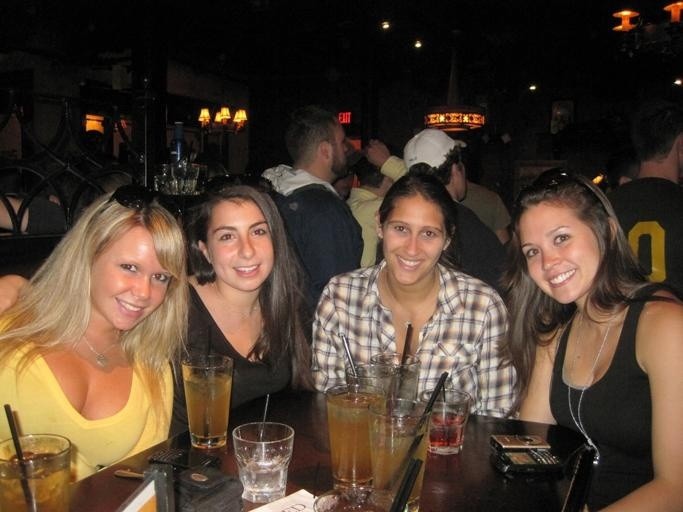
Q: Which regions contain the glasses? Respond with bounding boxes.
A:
[102,184,183,222]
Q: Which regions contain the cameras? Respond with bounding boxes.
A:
[491,434,551,454]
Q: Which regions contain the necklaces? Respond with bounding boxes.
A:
[567,311,617,467]
[384,277,436,329]
[82,336,122,367]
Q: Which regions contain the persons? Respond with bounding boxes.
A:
[310,163,520,422]
[496,167,683,512]
[0,173,316,437]
[0,182,191,504]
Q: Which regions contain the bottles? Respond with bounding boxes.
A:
[168,119,188,163]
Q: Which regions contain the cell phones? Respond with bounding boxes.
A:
[501,449,562,473]
[148,449,221,472]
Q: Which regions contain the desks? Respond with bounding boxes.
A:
[70,388,588,508]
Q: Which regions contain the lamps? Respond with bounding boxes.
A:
[422,106,487,133]
[610,1,683,64]
[196,106,249,137]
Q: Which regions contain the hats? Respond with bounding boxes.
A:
[403,127,467,173]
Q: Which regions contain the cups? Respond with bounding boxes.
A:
[417,387,472,454]
[182,355,234,449]
[314,486,410,512]
[0,432,73,511]
[325,352,431,512]
[232,421,296,504]
[153,161,209,198]
